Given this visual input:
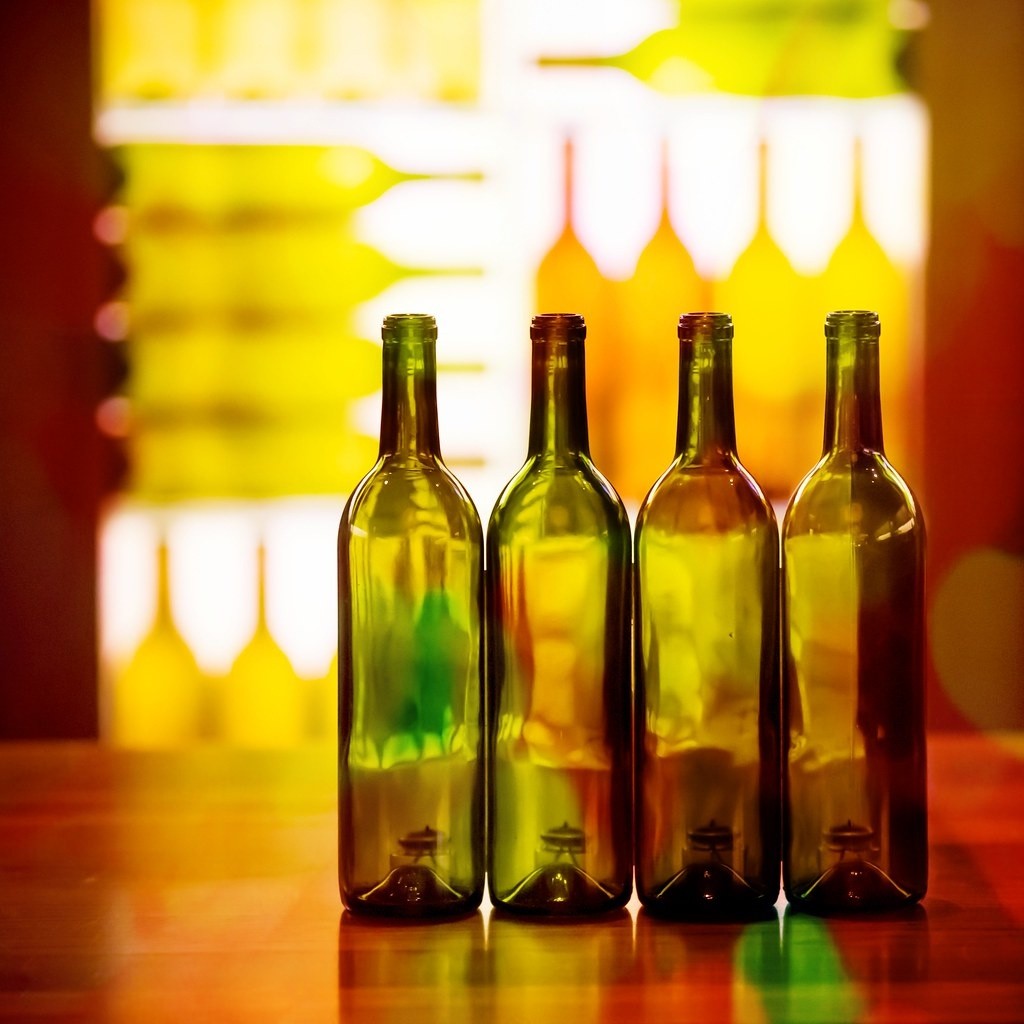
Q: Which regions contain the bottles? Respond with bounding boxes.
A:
[334,312,484,927]
[780,308,929,923]
[633,309,780,929]
[485,310,634,926]
